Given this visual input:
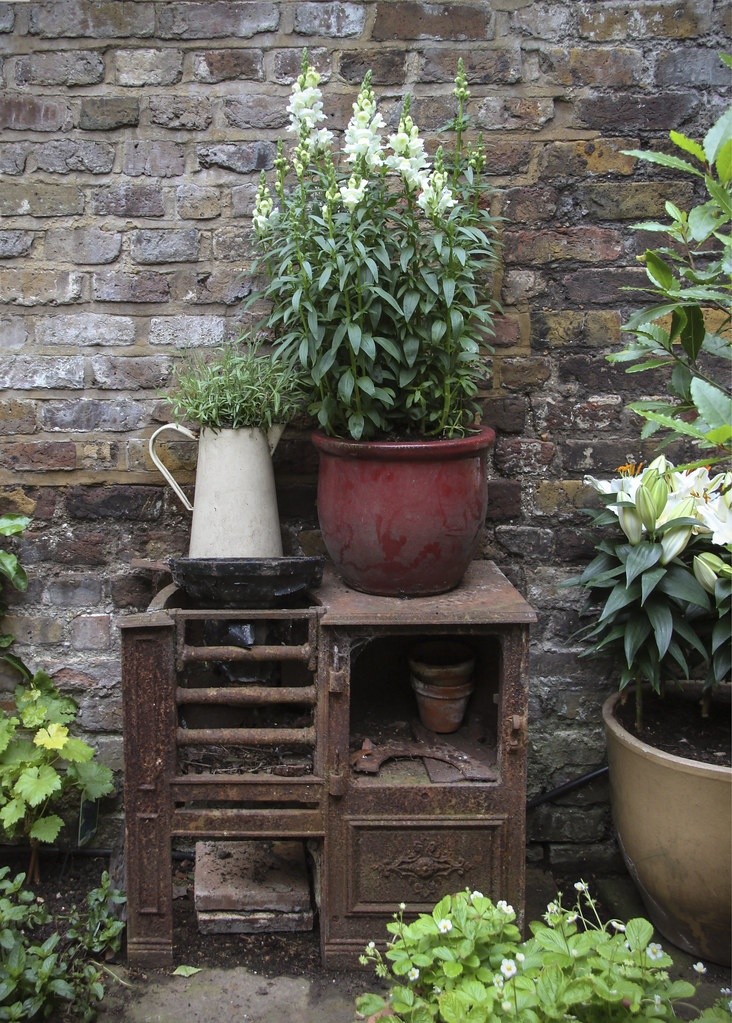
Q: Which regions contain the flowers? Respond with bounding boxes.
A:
[247,42,517,438]
[553,107,731,732]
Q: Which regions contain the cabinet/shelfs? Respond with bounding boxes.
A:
[119,560,538,979]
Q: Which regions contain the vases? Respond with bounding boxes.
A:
[600,681,732,971]
[303,424,497,601]
